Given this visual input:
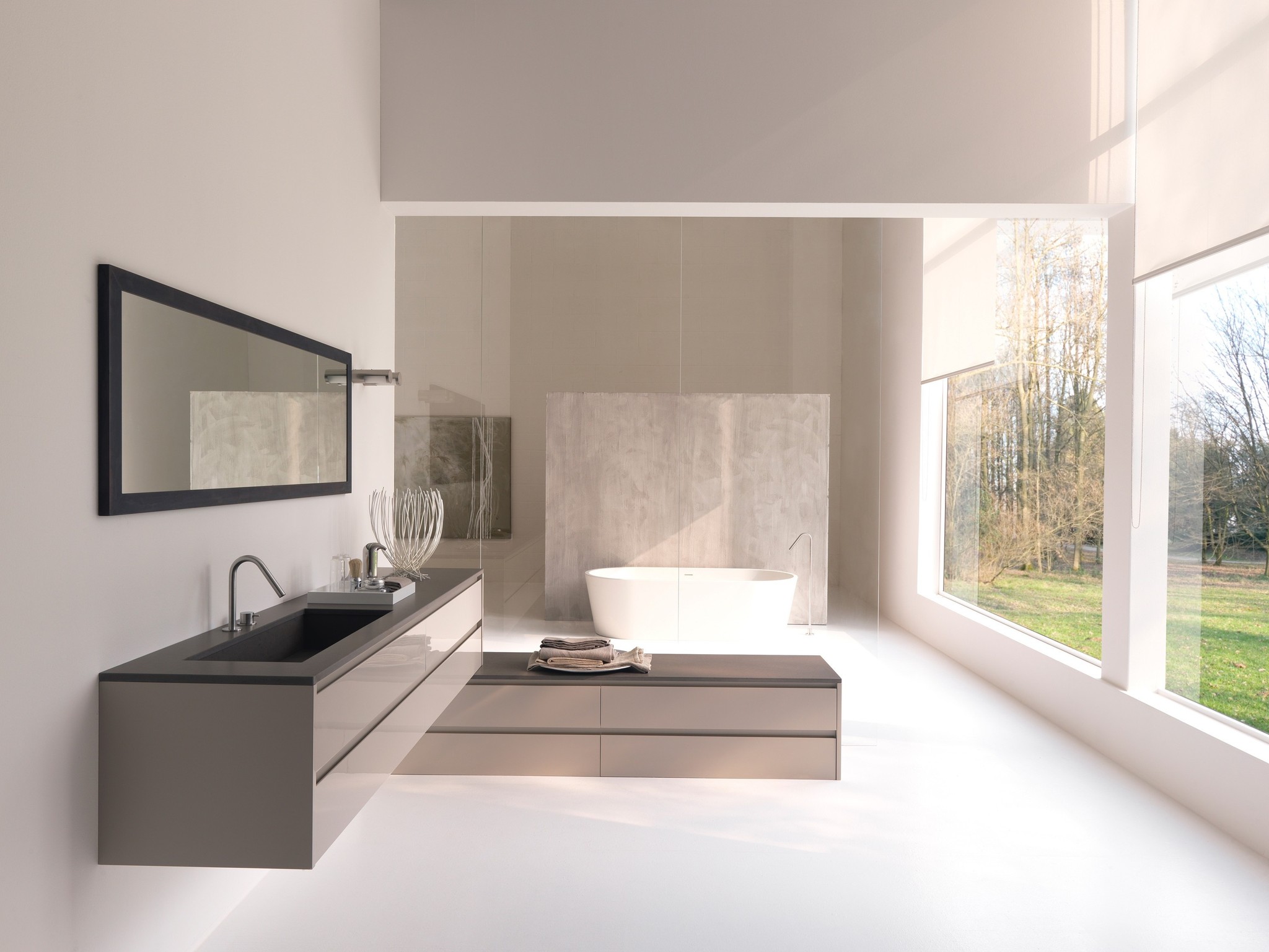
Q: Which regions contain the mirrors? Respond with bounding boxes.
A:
[97,264,352,516]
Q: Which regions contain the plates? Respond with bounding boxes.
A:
[540,666,631,672]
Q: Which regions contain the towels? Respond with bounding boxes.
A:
[526,635,653,674]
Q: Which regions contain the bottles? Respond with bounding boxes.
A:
[329,555,345,592]
[341,554,351,581]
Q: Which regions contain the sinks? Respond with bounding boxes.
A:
[182,608,395,663]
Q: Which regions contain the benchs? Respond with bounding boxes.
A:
[393,652,841,782]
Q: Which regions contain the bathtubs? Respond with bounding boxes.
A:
[584,565,797,638]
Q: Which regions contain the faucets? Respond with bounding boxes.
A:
[789,532,814,635]
[221,554,286,632]
